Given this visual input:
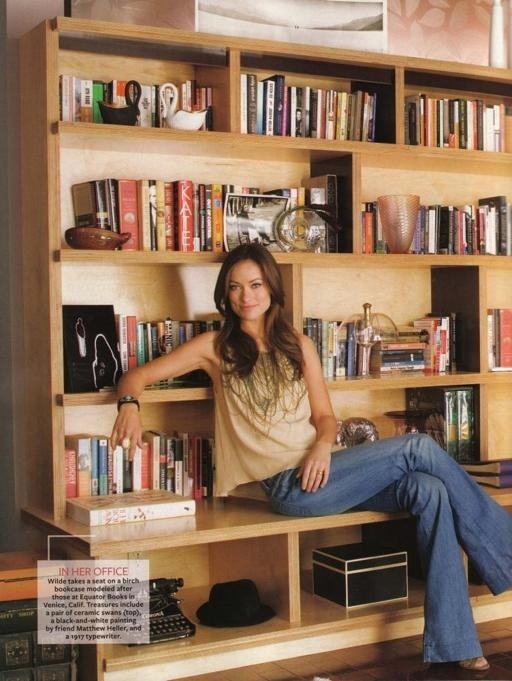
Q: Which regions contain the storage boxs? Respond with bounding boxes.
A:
[311,543,408,609]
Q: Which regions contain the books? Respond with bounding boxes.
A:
[66,488,195,524]
[487,309,510,371]
[63,304,226,393]
[405,387,480,464]
[361,196,508,256]
[72,174,349,253]
[67,430,214,501]
[303,311,466,378]
[240,73,396,144]
[404,93,509,153]
[60,73,213,132]
[461,459,511,490]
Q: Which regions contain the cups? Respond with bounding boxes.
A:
[377,194,420,253]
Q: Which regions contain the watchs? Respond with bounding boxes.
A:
[118,395,140,411]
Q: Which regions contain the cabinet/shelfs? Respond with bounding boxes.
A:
[12,18,511,681]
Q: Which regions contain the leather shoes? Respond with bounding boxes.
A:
[431,657,490,671]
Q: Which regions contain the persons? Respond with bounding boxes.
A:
[105,241,512,671]
[105,243,512,671]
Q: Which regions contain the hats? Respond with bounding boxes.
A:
[195,579,278,629]
